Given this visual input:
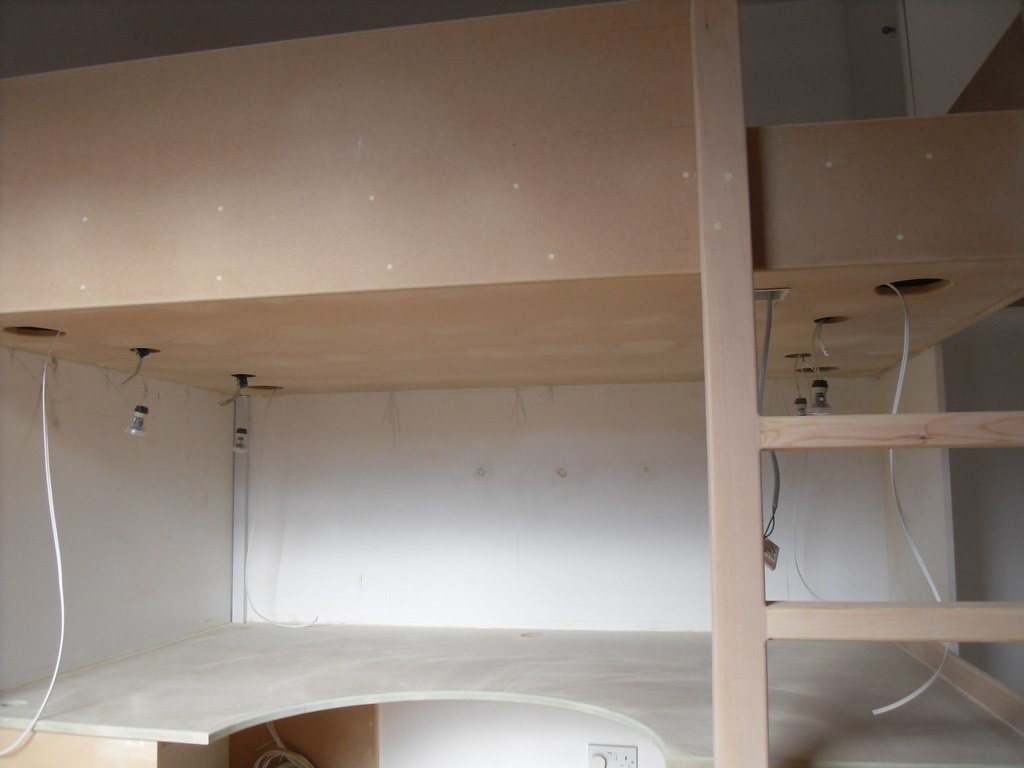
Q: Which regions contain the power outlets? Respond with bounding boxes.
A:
[587,744,638,768]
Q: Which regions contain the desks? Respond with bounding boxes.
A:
[0,622,1024,768]
